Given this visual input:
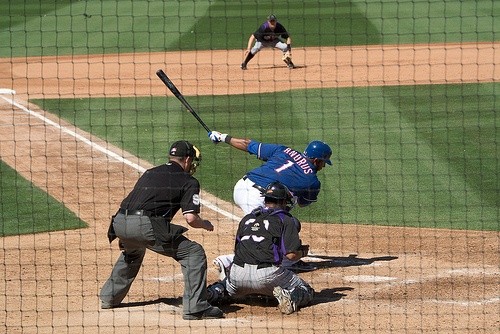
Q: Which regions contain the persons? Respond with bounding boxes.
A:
[209,131,333,284]
[241,15,294,69]
[207,181,315,314]
[99,142,224,320]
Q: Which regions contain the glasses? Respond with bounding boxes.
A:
[269,19,276,22]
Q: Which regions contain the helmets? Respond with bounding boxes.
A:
[262,180,294,199]
[169,140,202,160]
[303,140,333,166]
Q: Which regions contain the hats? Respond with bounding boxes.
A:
[268,15,276,21]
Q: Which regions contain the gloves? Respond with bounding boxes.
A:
[208,130,232,144]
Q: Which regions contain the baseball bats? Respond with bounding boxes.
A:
[156,68,218,145]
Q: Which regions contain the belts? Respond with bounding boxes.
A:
[119,208,151,217]
[233,261,272,271]
[243,175,267,193]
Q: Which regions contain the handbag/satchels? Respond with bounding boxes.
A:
[151,216,188,242]
[107,213,117,244]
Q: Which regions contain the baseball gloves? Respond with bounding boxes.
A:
[281,50,292,64]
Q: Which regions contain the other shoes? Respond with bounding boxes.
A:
[241,62,247,70]
[287,61,295,69]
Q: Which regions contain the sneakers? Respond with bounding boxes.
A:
[213,257,229,281]
[207,282,225,304]
[101,301,122,310]
[289,260,317,271]
[182,307,223,320]
[273,286,297,315]
